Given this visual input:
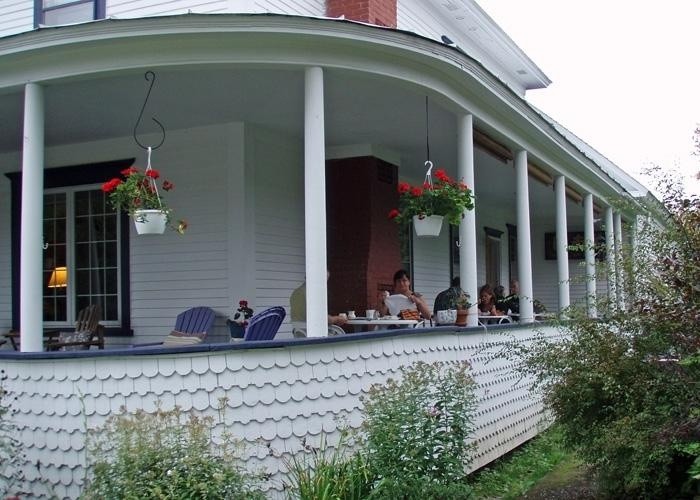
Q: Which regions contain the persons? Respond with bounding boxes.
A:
[289,267,347,339]
[478,281,508,326]
[377,269,433,330]
[507,279,519,313]
[433,276,460,317]
[495,286,507,303]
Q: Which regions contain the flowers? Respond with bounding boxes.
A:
[226,300,253,326]
[385,167,469,223]
[100,165,176,207]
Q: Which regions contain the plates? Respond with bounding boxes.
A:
[383,315,402,320]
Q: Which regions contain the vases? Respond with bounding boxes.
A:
[229,322,246,338]
[410,212,444,238]
[131,208,170,236]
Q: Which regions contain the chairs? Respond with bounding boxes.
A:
[129,306,215,347]
[289,321,346,339]
[0,304,104,352]
[243,306,286,341]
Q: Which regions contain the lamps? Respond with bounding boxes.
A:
[46,265,68,295]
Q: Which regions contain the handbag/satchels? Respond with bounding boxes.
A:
[401,309,421,320]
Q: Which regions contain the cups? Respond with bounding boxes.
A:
[365,309,376,319]
[507,308,512,315]
[348,310,356,319]
[339,312,347,319]
